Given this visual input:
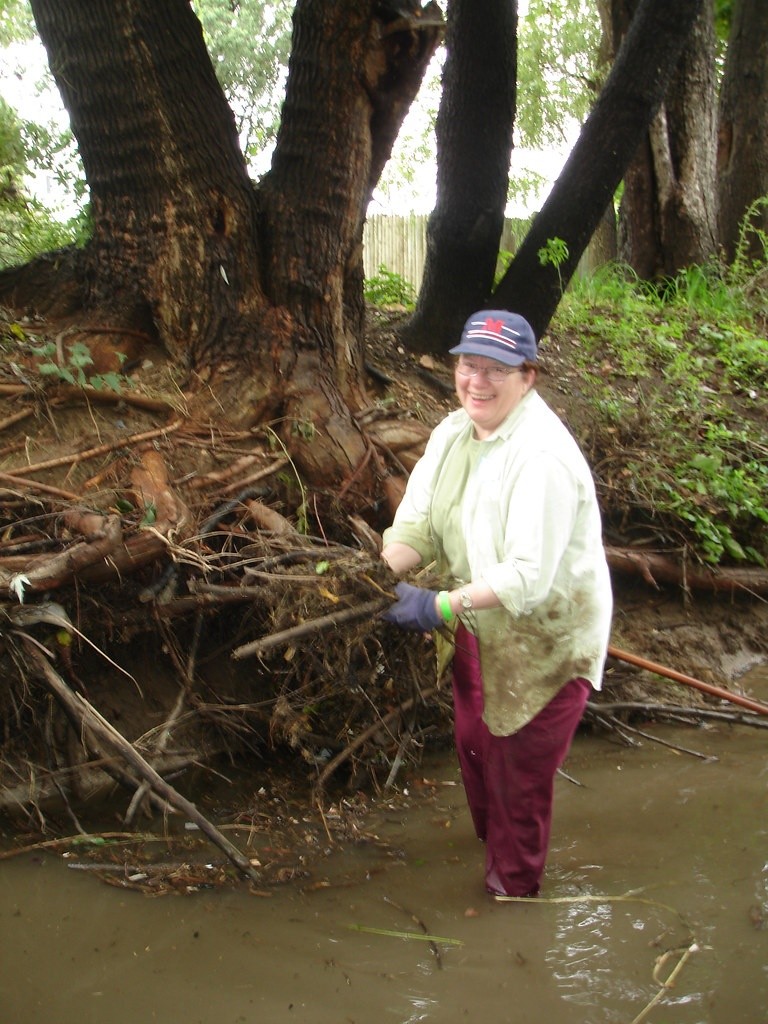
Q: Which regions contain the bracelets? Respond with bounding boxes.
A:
[438,590,454,623]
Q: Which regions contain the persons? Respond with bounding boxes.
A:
[377,307,613,902]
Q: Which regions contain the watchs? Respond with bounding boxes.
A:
[457,584,473,611]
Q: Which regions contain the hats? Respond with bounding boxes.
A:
[449,310,537,366]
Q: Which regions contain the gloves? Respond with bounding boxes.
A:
[381,582,443,632]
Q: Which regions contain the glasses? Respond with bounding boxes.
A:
[454,361,526,382]
